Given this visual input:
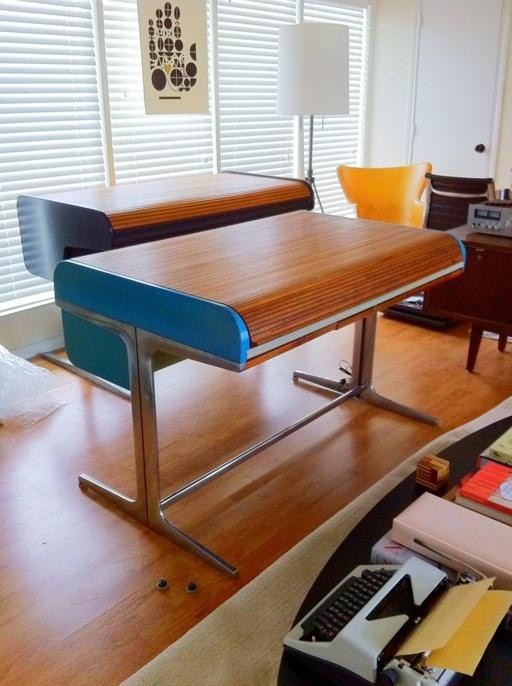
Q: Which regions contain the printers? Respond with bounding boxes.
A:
[282,555,458,686]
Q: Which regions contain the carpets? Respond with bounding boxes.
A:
[113,397,512,686]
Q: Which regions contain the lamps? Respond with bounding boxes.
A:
[279,23,349,211]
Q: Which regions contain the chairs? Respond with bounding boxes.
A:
[422,174,495,232]
[338,164,433,227]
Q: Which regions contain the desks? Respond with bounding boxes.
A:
[278,416,512,686]
[17,171,314,402]
[422,225,512,372]
[54,210,466,581]
